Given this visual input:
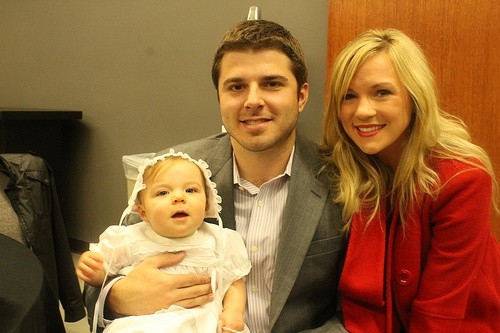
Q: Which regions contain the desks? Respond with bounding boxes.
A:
[1,104,83,217]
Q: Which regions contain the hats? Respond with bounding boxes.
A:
[119,150,224,228]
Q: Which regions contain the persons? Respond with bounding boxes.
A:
[322,26,500,333]
[75,154,250,333]
[81,20,366,332]
